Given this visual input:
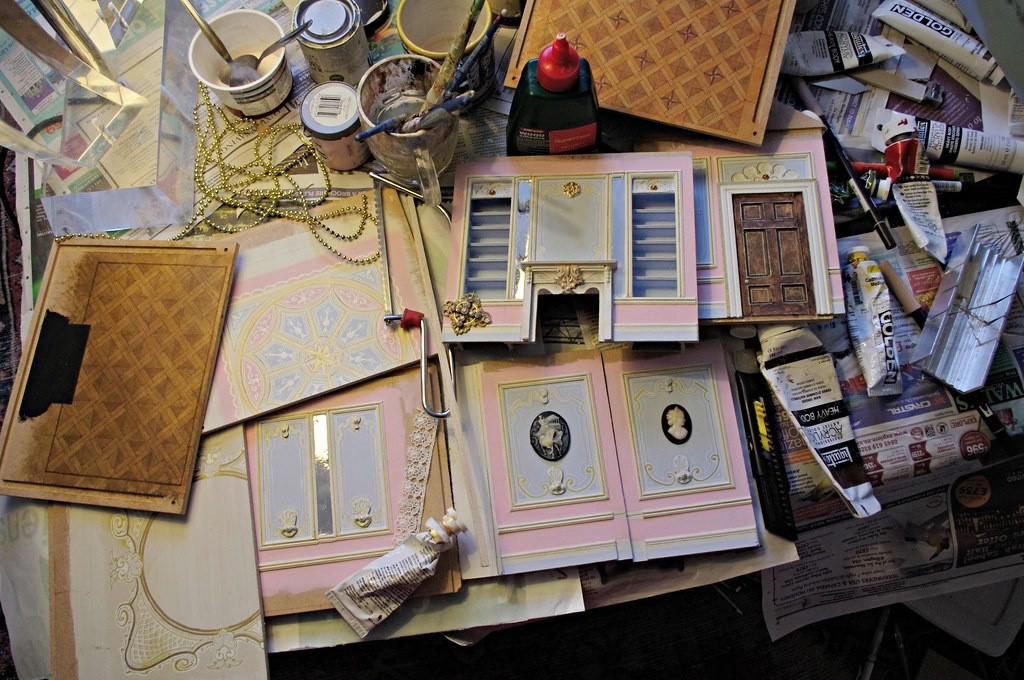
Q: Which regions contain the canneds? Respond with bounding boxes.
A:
[299,80,369,171]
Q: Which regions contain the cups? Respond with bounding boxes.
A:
[356,54,459,205]
[396,0,495,115]
[188,9,294,119]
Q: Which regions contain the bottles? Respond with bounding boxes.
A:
[299,80,371,172]
[505,33,601,156]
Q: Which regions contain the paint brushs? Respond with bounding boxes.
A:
[419,0,508,116]
[399,77,474,133]
[354,112,410,142]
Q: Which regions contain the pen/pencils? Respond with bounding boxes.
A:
[788,74,897,250]
[826,160,959,181]
[862,182,980,196]
[877,261,1016,455]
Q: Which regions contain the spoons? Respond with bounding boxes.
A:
[218,19,313,86]
[180,0,262,88]
